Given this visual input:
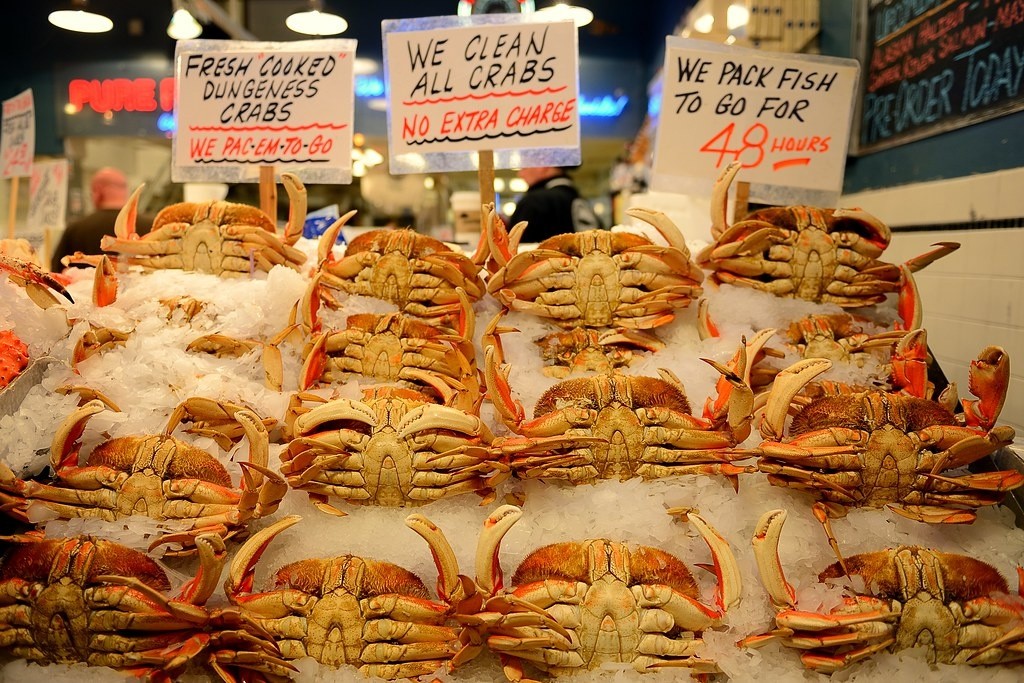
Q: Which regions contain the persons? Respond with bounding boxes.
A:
[52,166,150,274]
[498,151,604,244]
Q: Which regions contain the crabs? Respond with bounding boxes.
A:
[0,160,1024,682]
